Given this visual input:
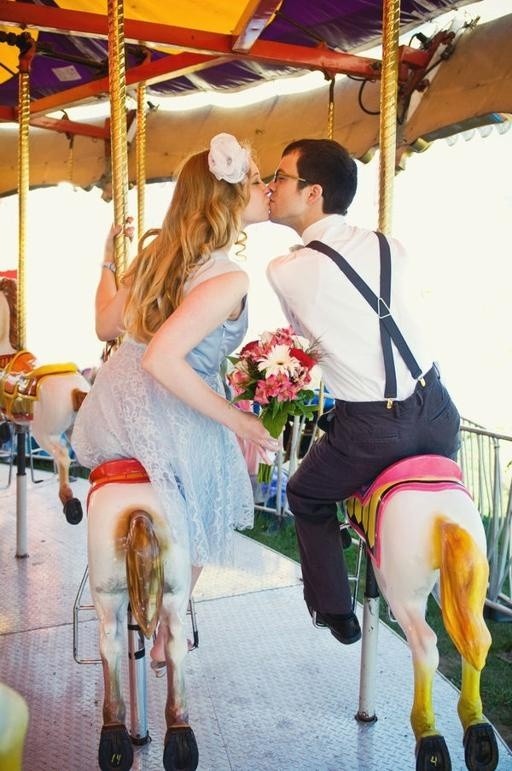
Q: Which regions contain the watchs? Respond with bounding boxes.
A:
[103,261,118,274]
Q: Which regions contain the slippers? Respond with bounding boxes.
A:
[150,639,194,678]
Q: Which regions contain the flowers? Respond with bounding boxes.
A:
[223,326,333,481]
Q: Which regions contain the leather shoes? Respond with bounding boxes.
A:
[302,595,363,644]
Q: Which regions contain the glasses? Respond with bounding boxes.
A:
[274,167,310,186]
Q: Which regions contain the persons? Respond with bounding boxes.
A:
[70,130,281,678]
[267,138,461,646]
